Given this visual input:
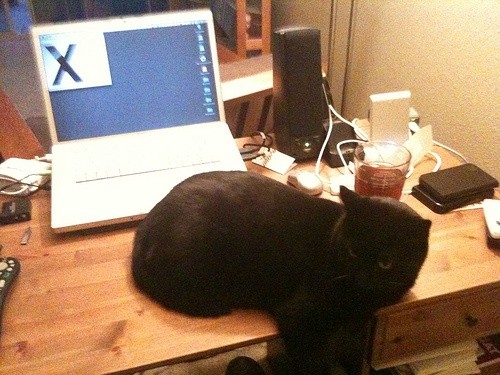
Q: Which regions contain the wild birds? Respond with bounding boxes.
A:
[30,9,248,235]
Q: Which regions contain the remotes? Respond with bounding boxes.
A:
[287,168,324,196]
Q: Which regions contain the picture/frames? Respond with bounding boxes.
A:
[1,119,500,375]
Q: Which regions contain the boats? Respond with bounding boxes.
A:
[273,26,324,163]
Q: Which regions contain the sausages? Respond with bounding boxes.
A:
[130,169,431,374]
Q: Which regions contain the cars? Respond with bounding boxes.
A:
[352,142,411,200]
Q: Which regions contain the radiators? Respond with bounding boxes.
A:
[0,257,20,324]
